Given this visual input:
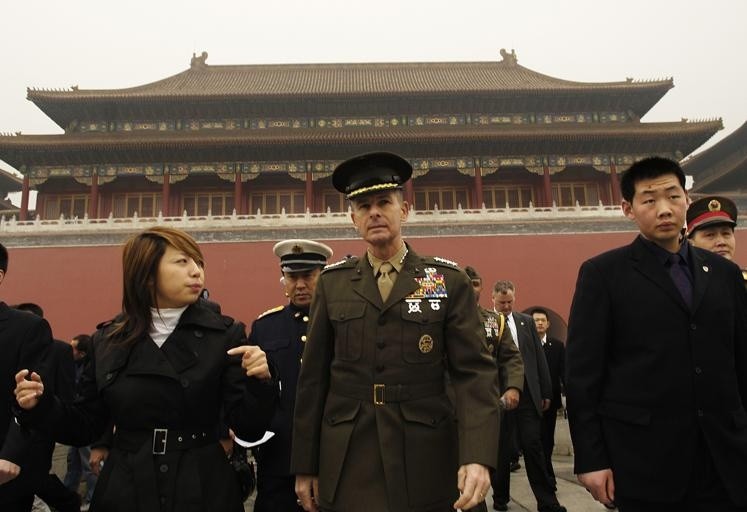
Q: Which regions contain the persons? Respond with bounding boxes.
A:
[289,151,501,511]
[221,240,333,512]
[564,157,745,512]
[0,245,110,512]
[686,195,746,280]
[13,226,278,511]
[464,266,566,512]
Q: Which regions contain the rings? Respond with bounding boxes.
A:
[479,492,484,498]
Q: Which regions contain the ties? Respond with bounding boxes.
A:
[504,315,509,325]
[669,253,693,312]
[377,262,394,303]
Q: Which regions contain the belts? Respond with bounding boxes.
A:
[116,418,221,454]
[329,378,441,405]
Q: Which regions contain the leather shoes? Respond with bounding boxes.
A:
[493,500,508,511]
[538,503,567,512]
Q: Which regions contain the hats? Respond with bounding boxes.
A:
[687,197,737,236]
[332,152,413,200]
[273,240,333,273]
